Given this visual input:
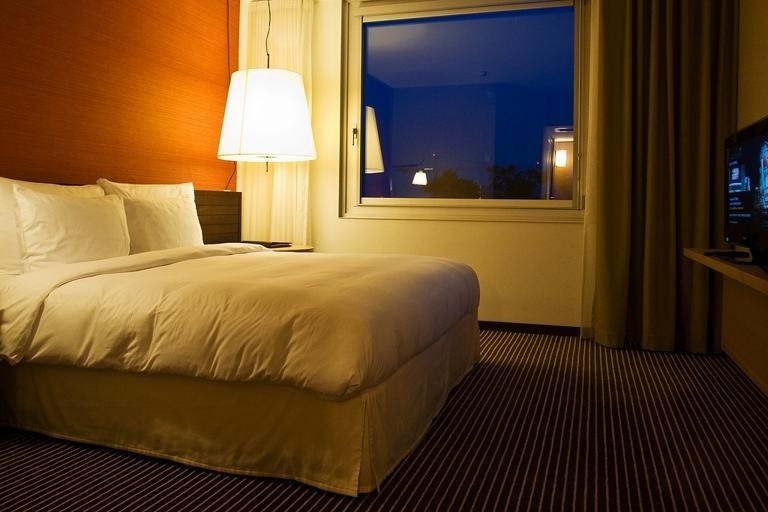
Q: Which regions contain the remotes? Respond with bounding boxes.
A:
[703,251,750,258]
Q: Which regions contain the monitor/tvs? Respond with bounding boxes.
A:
[723,114,768,273]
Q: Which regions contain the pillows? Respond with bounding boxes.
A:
[1,176,204,274]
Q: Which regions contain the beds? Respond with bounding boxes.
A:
[0,244,480,494]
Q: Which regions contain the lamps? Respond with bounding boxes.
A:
[365,105,384,175]
[216,1,317,173]
